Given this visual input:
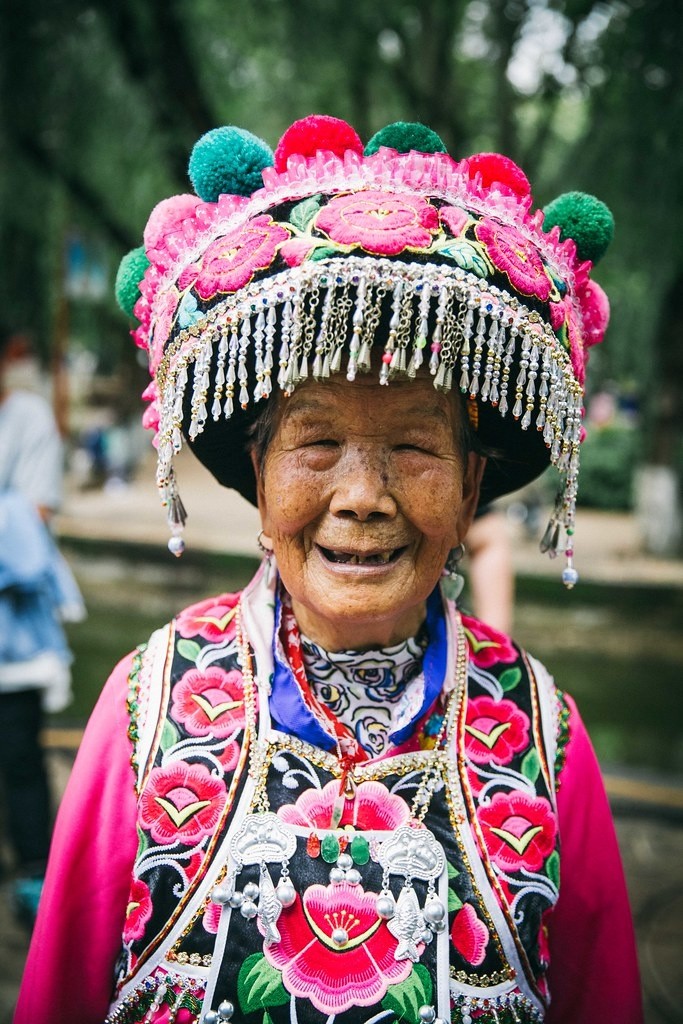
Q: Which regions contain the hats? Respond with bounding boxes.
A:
[116,115,613,589]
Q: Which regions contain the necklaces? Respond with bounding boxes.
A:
[206,599,471,963]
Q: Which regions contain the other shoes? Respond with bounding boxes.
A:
[15,877,44,922]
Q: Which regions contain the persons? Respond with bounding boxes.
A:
[13,113,644,1024]
[0,349,526,927]
